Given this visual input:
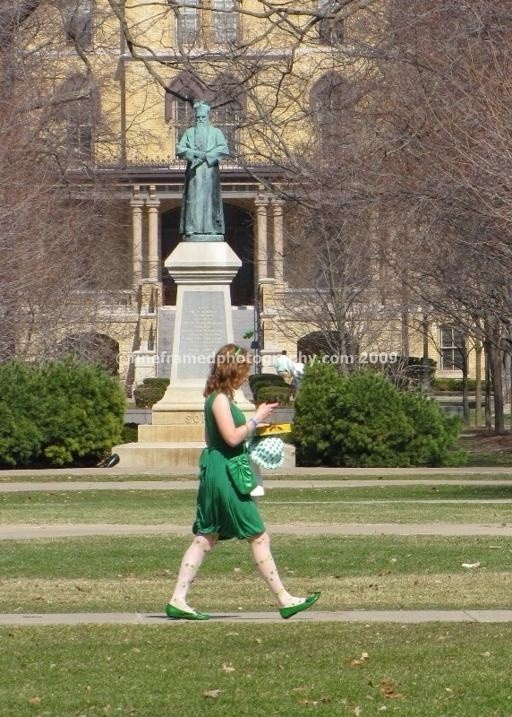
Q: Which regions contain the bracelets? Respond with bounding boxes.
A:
[246,422,252,433]
[250,417,258,427]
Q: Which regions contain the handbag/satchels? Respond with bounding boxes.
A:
[225,424,257,494]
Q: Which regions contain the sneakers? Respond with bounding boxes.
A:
[162,602,207,621]
[280,593,321,620]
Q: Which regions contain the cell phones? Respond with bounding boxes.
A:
[266,397,278,405]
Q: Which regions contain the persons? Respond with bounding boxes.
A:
[177,100,230,236]
[290,362,305,399]
[275,349,293,383]
[164,341,321,622]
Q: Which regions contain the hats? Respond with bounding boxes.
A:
[248,432,285,470]
